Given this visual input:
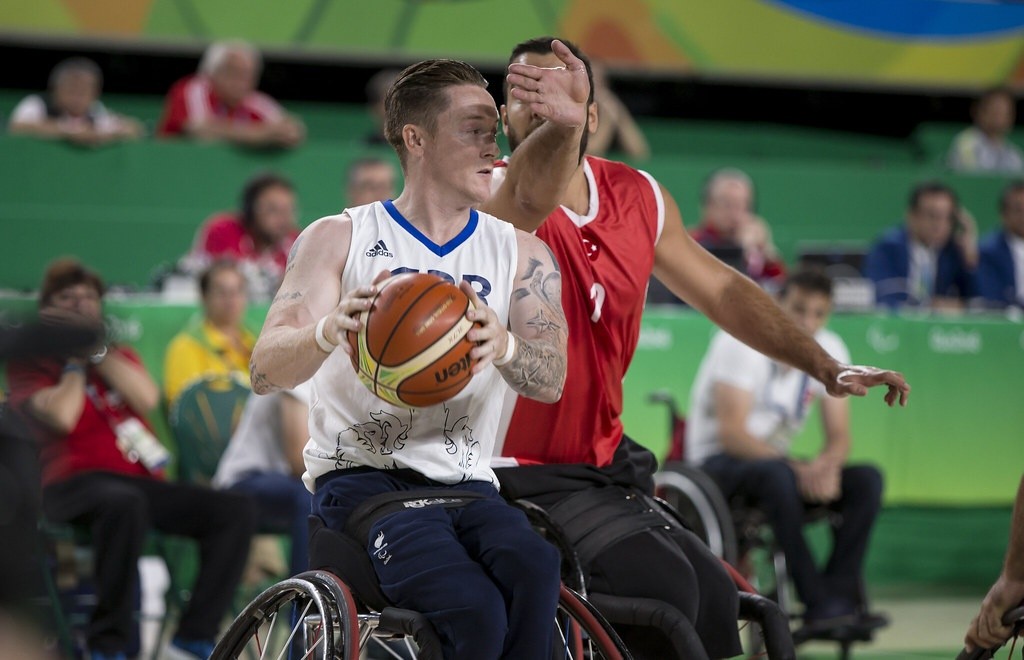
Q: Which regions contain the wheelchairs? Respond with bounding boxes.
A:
[651,390,851,659]
[205,488,795,660]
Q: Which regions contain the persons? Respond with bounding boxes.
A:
[164,263,312,660]
[9,53,138,147]
[686,172,783,283]
[479,35,910,659]
[964,473,1024,653]
[688,274,887,631]
[158,40,303,148]
[0,263,252,660]
[166,181,305,293]
[250,57,569,660]
[865,179,1024,316]
[948,87,1024,171]
[347,159,393,206]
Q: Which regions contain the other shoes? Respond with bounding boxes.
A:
[804,600,855,629]
[166,633,216,660]
[843,600,887,632]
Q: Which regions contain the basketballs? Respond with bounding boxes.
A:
[347,271,487,410]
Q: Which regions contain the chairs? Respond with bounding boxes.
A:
[18,370,300,660]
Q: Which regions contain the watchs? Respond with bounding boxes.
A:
[92,345,108,364]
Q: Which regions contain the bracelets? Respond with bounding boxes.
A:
[493,331,515,365]
[64,364,86,376]
[316,315,337,353]
